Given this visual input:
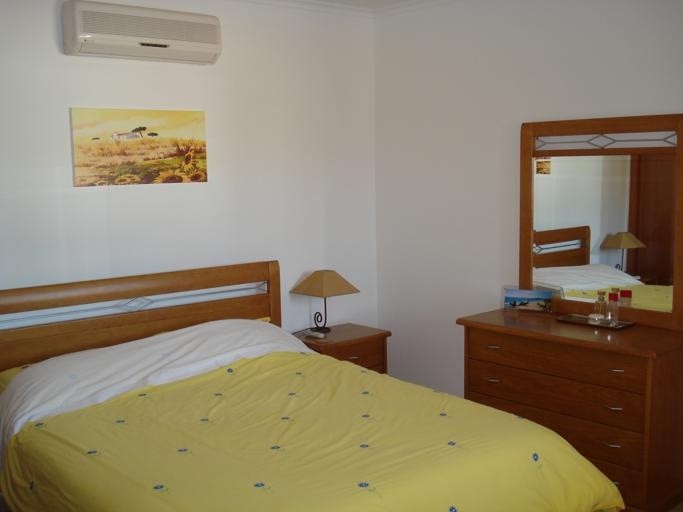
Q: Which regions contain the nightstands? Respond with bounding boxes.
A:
[297,322,392,376]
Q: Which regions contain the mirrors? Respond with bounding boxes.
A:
[519,112,683,333]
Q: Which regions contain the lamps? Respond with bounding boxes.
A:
[602,230,647,281]
[289,269,363,334]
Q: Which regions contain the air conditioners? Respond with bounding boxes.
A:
[60,0,223,65]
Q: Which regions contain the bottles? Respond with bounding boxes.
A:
[594,290,607,314]
[619,290,632,308]
[607,292,619,326]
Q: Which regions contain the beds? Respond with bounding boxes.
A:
[1,259,623,511]
[530,224,674,311]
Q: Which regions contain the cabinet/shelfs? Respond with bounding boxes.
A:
[454,308,683,511]
